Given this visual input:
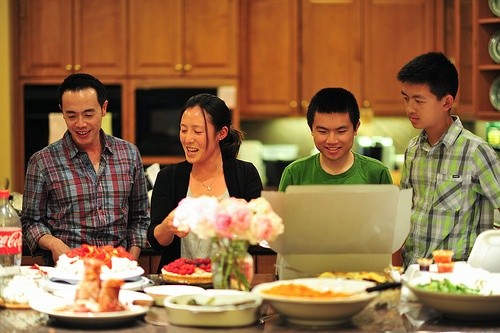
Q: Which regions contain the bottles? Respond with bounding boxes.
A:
[0,189,23,275]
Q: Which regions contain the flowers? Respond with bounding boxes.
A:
[174,196,286,289]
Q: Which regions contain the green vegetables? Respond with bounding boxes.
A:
[418,278,494,296]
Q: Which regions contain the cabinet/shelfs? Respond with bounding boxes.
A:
[17,0,127,84]
[366,0,473,122]
[19,255,278,276]
[240,0,366,116]
[128,0,240,87]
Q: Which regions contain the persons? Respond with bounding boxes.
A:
[276,87,404,267]
[396,51,500,274]
[20,74,150,261]
[147,95,277,276]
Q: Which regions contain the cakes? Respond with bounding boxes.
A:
[54,244,144,280]
[161,256,213,283]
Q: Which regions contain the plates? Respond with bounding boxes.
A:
[164,288,262,327]
[403,270,500,318]
[47,274,222,288]
[28,282,154,323]
[253,277,380,326]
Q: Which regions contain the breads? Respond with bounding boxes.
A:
[72,257,128,312]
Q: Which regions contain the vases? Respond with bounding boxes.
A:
[210,238,254,291]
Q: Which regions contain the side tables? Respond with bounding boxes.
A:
[472,0,500,121]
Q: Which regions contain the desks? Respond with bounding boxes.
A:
[0,275,500,333]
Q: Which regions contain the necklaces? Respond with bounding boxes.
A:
[194,171,216,191]
